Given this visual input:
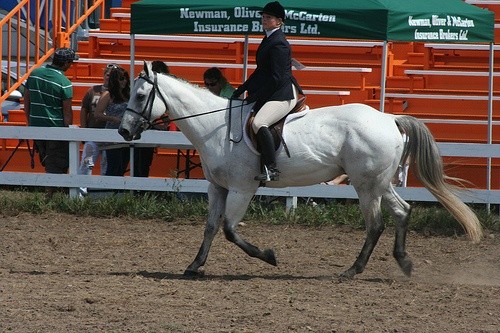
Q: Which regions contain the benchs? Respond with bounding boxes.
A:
[0,0,413,184]
[384,0,500,190]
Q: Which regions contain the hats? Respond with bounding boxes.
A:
[54,47,80,60]
[258,1,285,21]
[104,62,120,75]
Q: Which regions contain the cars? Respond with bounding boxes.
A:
[2,60,46,124]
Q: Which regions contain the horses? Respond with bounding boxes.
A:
[118,61,486,278]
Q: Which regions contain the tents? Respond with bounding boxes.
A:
[130,0,494,217]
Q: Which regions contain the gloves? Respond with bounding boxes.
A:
[244,92,258,105]
[229,88,242,100]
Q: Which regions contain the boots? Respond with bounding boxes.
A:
[254,126,279,181]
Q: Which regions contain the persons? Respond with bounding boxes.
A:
[203,67,239,99]
[79,60,169,199]
[229,1,305,182]
[24,47,80,196]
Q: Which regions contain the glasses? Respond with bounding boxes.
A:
[117,76,129,81]
[205,79,222,86]
